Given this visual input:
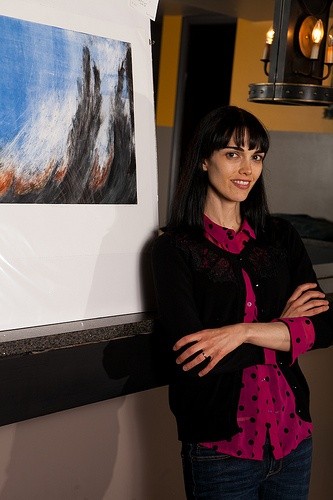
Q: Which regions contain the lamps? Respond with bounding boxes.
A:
[246,0,275,104]
[273,0,333,107]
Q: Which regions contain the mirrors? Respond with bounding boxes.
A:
[147,0,333,279]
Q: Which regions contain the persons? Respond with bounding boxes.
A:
[138,104,333,500]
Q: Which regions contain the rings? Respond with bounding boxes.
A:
[202,352,208,359]
